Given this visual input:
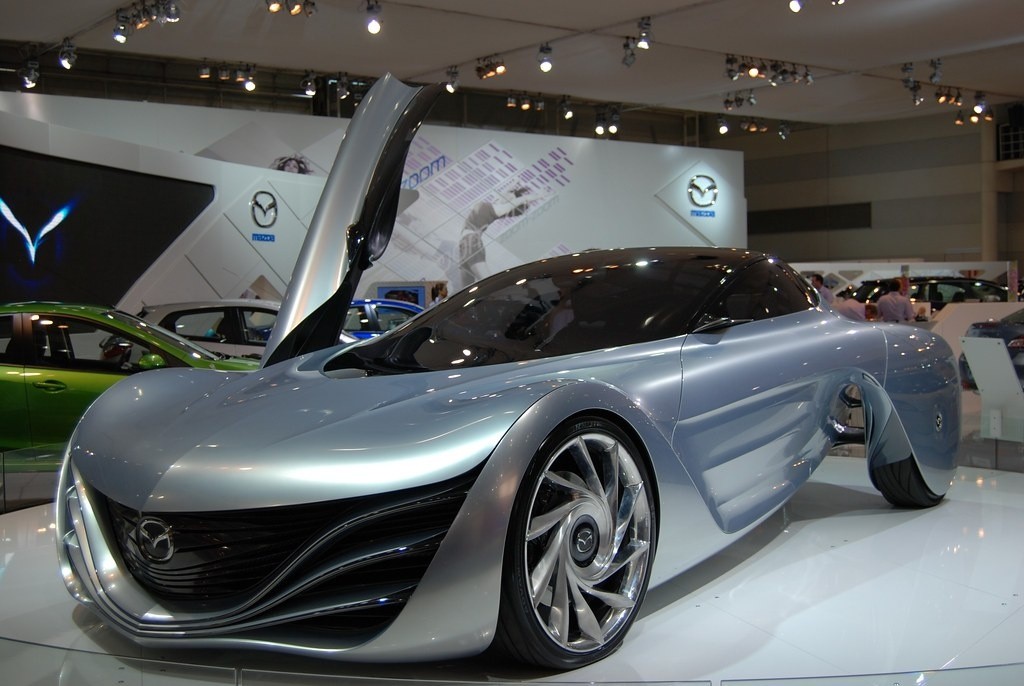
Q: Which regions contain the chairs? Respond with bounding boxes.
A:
[48,318,77,360]
[4,327,47,364]
[911,293,917,299]
[932,292,943,301]
[951,291,966,302]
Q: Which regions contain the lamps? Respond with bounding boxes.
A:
[723,89,758,112]
[20,60,40,89]
[714,114,729,136]
[365,0,382,35]
[110,0,181,44]
[535,92,546,113]
[445,65,460,94]
[196,58,211,79]
[637,16,652,50]
[789,0,846,13]
[725,53,815,87]
[929,58,944,85]
[621,36,637,67]
[266,0,318,19]
[594,102,623,136]
[506,89,517,109]
[910,81,925,108]
[559,95,575,121]
[336,72,351,100]
[217,61,230,81]
[899,63,915,89]
[969,91,994,124]
[953,110,965,127]
[520,91,532,111]
[475,53,509,80]
[302,69,317,96]
[536,42,552,74]
[777,120,792,141]
[59,38,78,71]
[233,62,257,93]
[935,86,965,107]
[740,116,769,133]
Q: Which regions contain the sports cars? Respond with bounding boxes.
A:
[49,71,963,673]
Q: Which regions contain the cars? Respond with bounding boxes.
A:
[850,269,1024,396]
[0,297,470,475]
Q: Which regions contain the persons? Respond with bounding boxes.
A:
[810,273,915,323]
[428,283,449,307]
